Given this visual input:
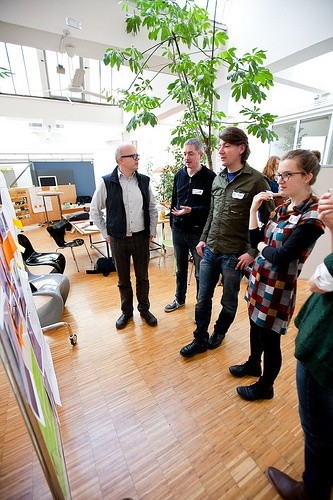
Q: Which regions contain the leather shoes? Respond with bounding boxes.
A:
[266,466,305,500]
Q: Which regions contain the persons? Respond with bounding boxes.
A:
[230,149,332,500]
[90,145,159,329]
[164,139,217,323]
[180,128,276,358]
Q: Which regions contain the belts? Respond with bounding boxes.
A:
[124,231,146,237]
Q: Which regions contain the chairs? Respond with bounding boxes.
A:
[17,196,93,328]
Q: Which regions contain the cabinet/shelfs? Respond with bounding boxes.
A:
[8,184,76,227]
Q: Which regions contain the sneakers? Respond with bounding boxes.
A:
[116,313,133,329]
[180,340,208,357]
[208,332,225,350]
[164,300,185,311]
[140,311,157,326]
[237,376,274,401]
[194,304,196,324]
[229,356,262,377]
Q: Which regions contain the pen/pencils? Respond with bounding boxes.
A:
[81,224,91,230]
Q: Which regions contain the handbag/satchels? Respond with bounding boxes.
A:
[86,258,117,276]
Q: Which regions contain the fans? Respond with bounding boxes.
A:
[42,68,109,101]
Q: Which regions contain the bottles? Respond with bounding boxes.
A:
[160,206,165,220]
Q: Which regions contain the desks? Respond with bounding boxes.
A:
[62,212,169,259]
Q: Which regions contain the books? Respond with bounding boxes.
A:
[12,197,28,207]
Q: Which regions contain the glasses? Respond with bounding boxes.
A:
[272,171,306,181]
[121,154,140,160]
